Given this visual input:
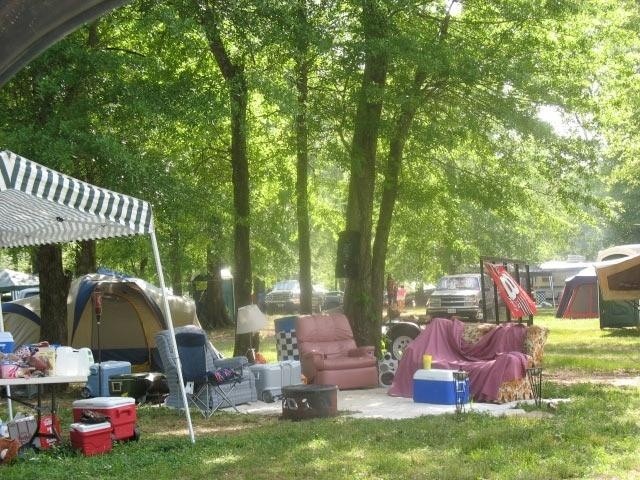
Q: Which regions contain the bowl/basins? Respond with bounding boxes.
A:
[0,363,17,380]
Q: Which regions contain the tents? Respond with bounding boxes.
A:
[0,267,226,371]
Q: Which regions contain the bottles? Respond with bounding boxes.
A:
[377,336,388,359]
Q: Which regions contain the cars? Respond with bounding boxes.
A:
[426,273,500,323]
[263,280,344,315]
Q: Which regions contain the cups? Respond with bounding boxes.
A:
[422,354,432,371]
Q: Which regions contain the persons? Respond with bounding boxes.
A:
[396,284,407,313]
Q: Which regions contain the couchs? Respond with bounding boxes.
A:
[399,318,550,405]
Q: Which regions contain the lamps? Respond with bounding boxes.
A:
[234,303,268,365]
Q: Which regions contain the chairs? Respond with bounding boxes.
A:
[155,324,257,422]
[296,312,380,391]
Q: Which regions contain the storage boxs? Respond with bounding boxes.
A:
[249,361,301,403]
[410,368,471,405]
[69,360,147,456]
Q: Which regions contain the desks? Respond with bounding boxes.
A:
[0,376,90,452]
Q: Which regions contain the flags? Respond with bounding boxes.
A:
[484,261,539,319]
